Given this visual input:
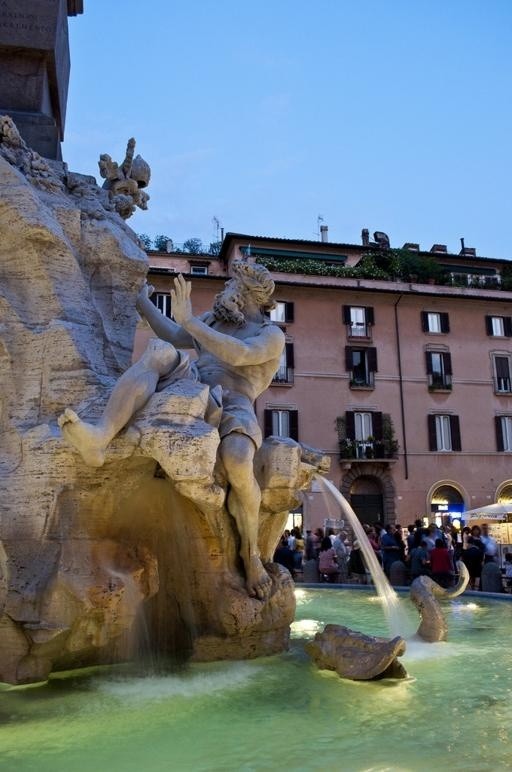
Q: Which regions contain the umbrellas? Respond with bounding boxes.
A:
[462,500,510,526]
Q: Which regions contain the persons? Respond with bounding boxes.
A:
[56,256,287,603]
[275,510,511,592]
